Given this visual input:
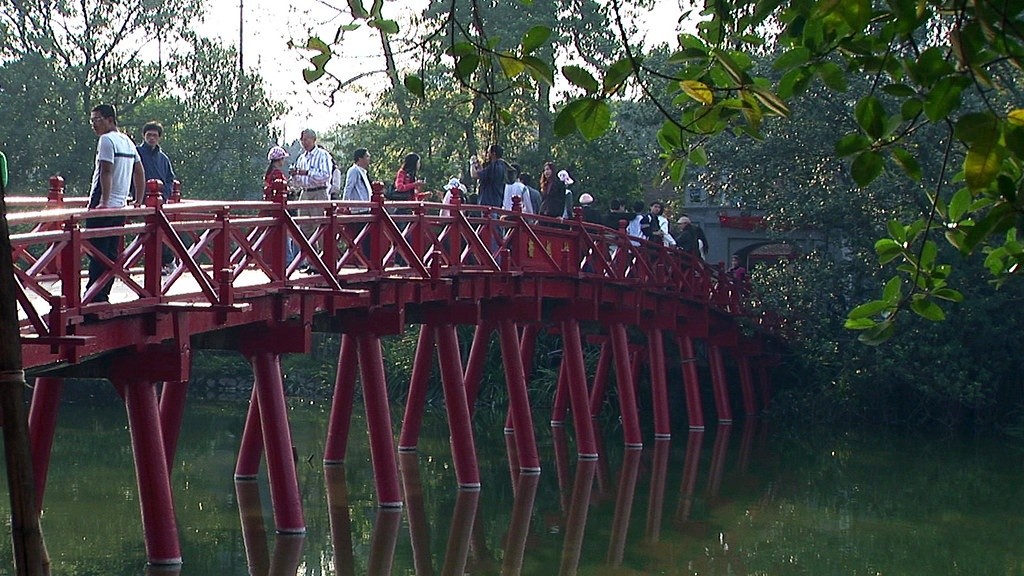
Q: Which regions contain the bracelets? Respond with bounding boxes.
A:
[470,162,475,166]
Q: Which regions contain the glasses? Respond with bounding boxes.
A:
[90,116,105,124]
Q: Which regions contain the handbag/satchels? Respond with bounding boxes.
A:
[384,176,412,201]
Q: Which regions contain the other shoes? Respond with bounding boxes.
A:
[162,264,178,276]
[300,268,320,275]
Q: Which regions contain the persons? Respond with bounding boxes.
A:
[500,161,574,249]
[129,121,178,276]
[258,146,294,269]
[385,152,433,267]
[83,104,146,304]
[602,198,708,277]
[725,255,746,285]
[470,144,517,267]
[578,193,602,273]
[288,129,341,275]
[438,177,467,253]
[342,147,374,265]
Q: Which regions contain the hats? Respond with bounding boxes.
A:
[579,192,593,204]
[268,147,291,160]
[443,177,460,191]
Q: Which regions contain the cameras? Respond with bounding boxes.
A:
[473,158,480,165]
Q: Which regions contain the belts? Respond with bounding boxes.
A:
[300,186,327,192]
